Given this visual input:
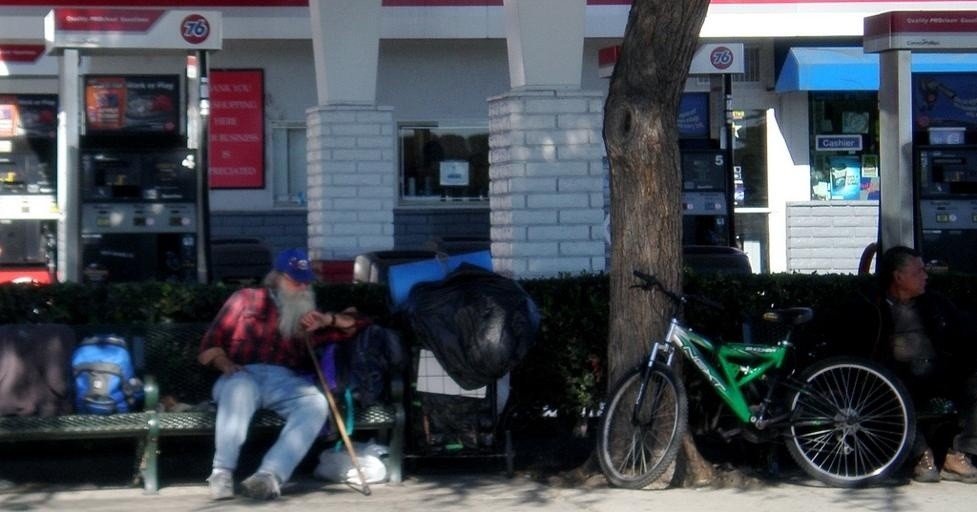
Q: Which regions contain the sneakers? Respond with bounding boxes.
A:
[901,445,941,483]
[208,468,235,499]
[939,450,977,484]
[239,471,280,501]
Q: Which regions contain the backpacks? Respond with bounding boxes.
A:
[70,324,147,416]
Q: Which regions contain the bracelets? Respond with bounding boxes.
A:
[329,312,335,327]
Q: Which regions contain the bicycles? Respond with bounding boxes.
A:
[595,270,918,490]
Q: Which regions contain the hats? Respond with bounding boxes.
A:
[273,247,320,283]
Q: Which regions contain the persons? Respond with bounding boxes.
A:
[195,246,377,498]
[850,245,976,483]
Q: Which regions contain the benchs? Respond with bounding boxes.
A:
[741,317,975,480]
[0,316,405,491]
